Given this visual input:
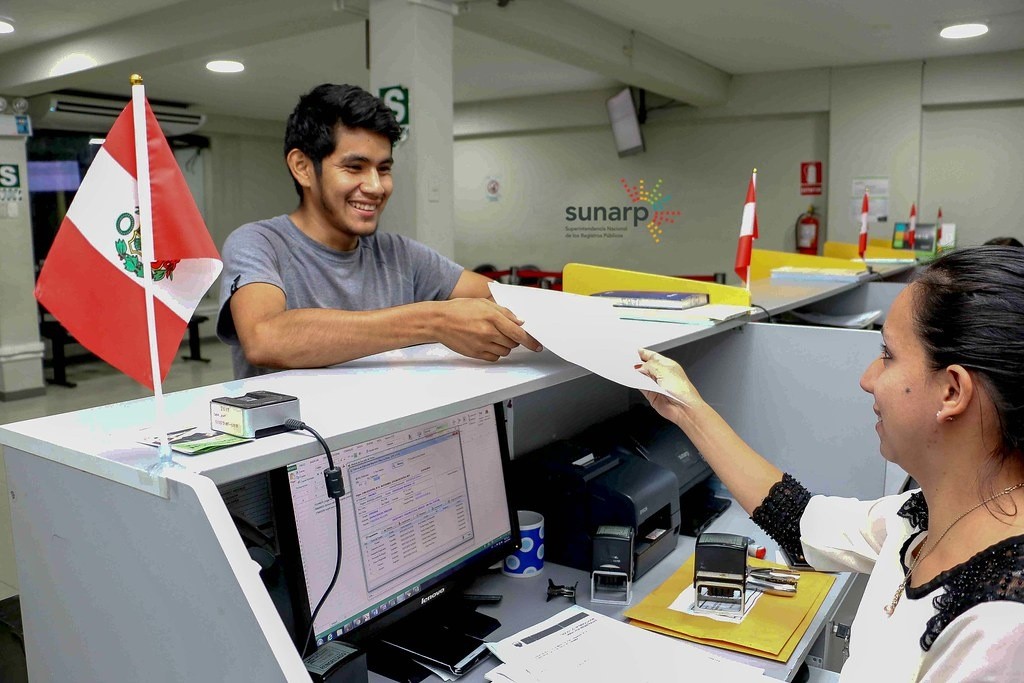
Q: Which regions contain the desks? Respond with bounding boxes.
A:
[0,251,946,683]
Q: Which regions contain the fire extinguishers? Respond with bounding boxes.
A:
[793,206,822,256]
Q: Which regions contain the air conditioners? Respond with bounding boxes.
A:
[25,95,206,138]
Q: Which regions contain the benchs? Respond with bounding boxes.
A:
[39,302,211,388]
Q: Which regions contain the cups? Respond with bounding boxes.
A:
[501,512,545,578]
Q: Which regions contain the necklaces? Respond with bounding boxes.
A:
[886,482,1024,614]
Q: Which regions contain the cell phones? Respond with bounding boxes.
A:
[378,617,487,671]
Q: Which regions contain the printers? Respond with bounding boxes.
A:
[505,402,731,583]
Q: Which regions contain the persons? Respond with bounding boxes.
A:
[637,244,1024,683]
[215,82,544,380]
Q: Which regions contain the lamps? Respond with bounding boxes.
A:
[0,97,32,137]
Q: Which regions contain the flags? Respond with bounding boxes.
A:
[908,202,917,247]
[33,75,223,389]
[734,168,759,284]
[859,189,869,257]
[937,207,942,241]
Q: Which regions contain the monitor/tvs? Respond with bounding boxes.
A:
[606,86,646,157]
[269,401,522,683]
